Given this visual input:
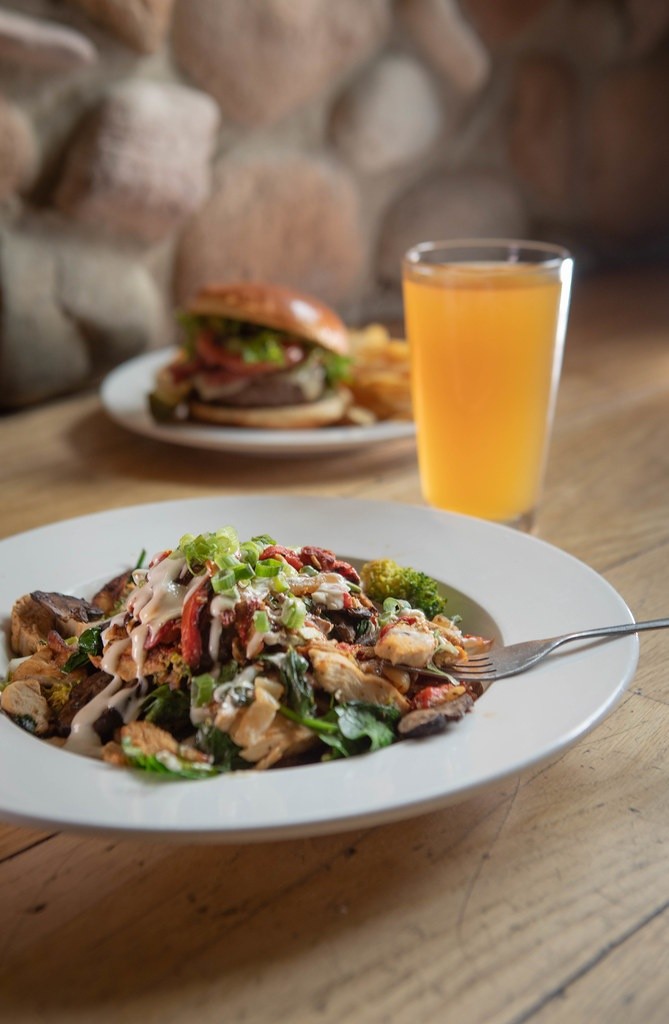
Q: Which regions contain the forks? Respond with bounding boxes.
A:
[442,618,669,683]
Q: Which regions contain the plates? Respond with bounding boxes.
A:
[98,343,415,455]
[0,492,644,847]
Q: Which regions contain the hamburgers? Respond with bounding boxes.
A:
[150,280,353,430]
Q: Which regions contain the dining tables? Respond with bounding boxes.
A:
[2,250,668,1024]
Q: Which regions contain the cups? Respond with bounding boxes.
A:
[398,238,575,536]
